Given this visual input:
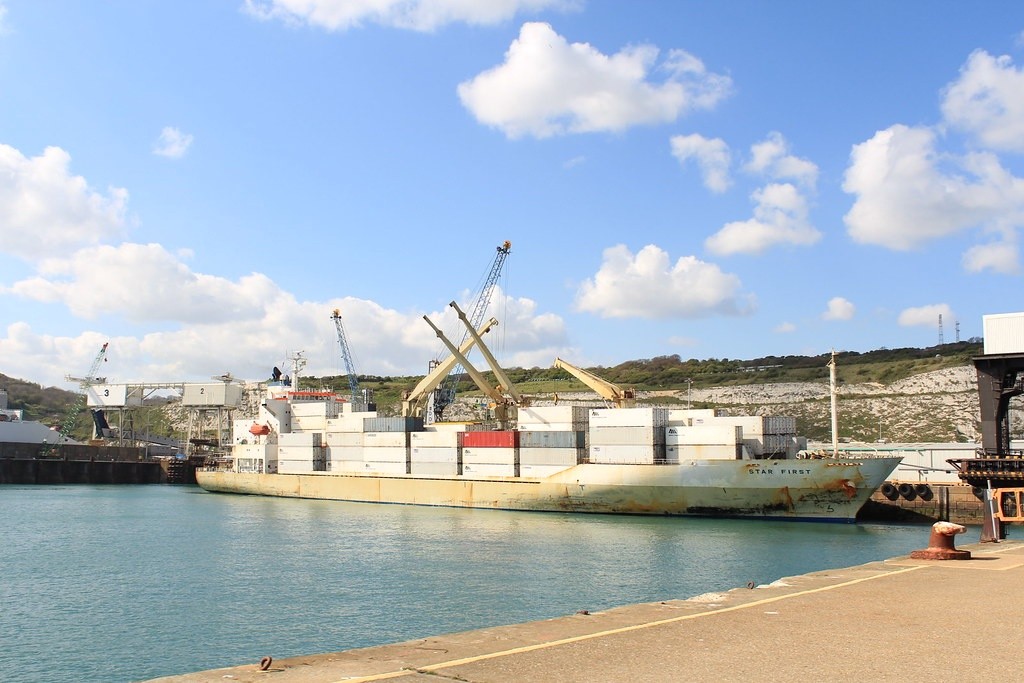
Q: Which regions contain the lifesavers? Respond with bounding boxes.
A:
[973,486,984,496]
[881,484,895,497]
[916,484,929,497]
[898,483,911,497]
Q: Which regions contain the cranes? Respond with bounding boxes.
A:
[38,342,107,460]
[329,308,376,411]
[425,240,513,424]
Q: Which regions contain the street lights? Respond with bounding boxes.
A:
[685,378,694,410]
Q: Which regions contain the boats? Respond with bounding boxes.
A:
[195,349,903,524]
